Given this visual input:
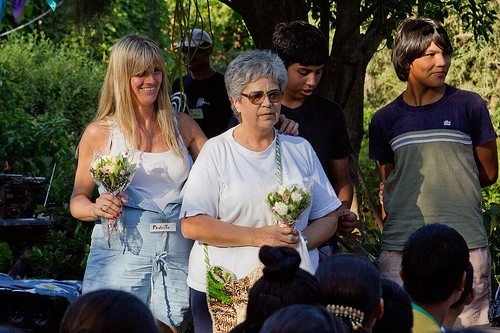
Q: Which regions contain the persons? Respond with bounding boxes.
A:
[71,35,207,333]
[176,51,342,333]
[0,0,500,333]
[369,16,499,331]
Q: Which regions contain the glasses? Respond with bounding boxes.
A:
[241,87,284,105]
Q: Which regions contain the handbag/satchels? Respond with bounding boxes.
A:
[206,263,266,333]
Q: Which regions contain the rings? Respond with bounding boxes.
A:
[101,204,105,212]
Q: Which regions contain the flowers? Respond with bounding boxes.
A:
[266,183,314,228]
[89,147,138,248]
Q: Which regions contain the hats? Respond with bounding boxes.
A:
[174,28,214,50]
